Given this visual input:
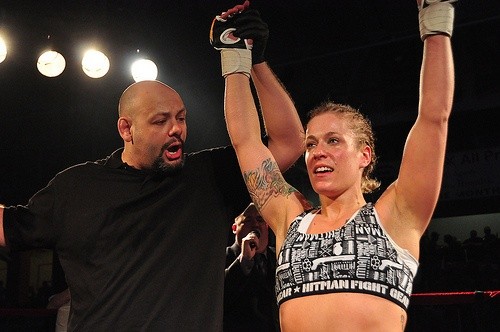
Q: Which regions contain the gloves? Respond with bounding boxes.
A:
[228,9,269,66]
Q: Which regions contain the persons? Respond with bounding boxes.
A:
[224,202,280,332]
[210,1,454,331]
[420,226,500,277]
[0,0,305,332]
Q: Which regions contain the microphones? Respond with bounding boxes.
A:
[250,228,261,248]
